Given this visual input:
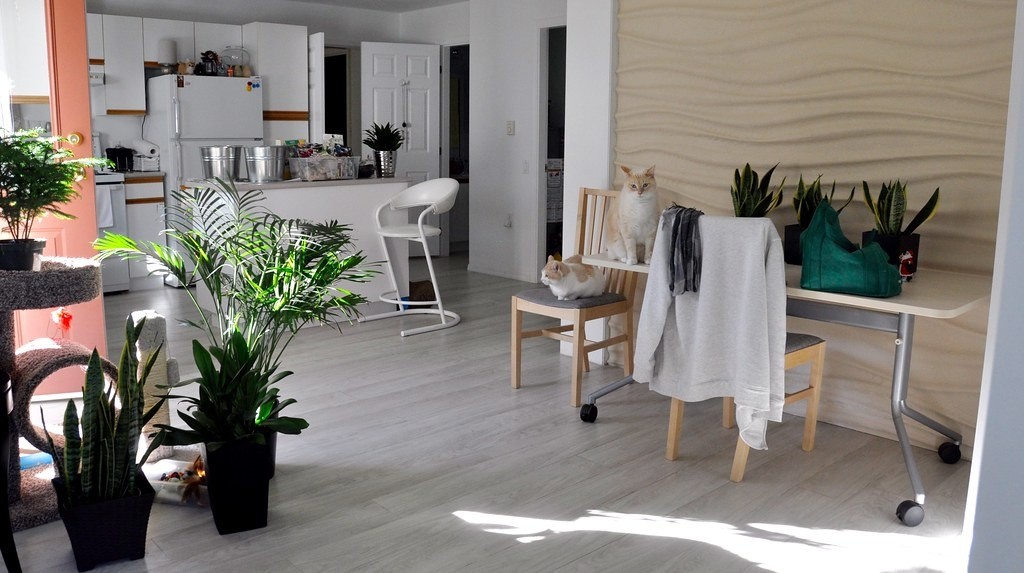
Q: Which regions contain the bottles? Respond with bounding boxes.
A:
[221,44,249,66]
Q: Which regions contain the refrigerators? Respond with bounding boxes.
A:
[142,73,264,273]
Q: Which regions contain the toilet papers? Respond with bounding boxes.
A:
[134,139,160,157]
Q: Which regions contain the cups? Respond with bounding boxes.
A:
[178,57,252,77]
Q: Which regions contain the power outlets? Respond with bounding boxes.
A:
[503,211,512,228]
[29,121,45,130]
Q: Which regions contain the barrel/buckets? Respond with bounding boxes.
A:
[244,144,285,183]
[199,145,241,180]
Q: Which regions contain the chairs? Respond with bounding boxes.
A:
[511,187,638,408]
[630,201,826,482]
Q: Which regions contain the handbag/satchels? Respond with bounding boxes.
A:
[799,194,903,298]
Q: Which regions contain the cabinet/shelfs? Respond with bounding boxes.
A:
[124,176,167,278]
[262,120,309,146]
[86,13,104,59]
[102,14,146,111]
[194,22,241,65]
[242,21,309,113]
[142,17,195,68]
[0,0,50,97]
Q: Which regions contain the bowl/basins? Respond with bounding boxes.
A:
[357,165,374,179]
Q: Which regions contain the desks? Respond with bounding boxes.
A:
[580,253,992,528]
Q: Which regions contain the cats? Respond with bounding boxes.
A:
[540,255,605,301]
[604,162,661,266]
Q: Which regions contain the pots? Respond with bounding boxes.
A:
[105,145,136,173]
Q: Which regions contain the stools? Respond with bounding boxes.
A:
[357,178,460,337]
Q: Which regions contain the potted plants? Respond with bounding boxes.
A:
[362,121,405,178]
[783,172,855,266]
[40,312,173,573]
[0,126,116,272]
[861,179,940,283]
[90,169,390,479]
[147,328,310,537]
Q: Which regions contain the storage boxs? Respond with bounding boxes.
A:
[288,156,360,182]
